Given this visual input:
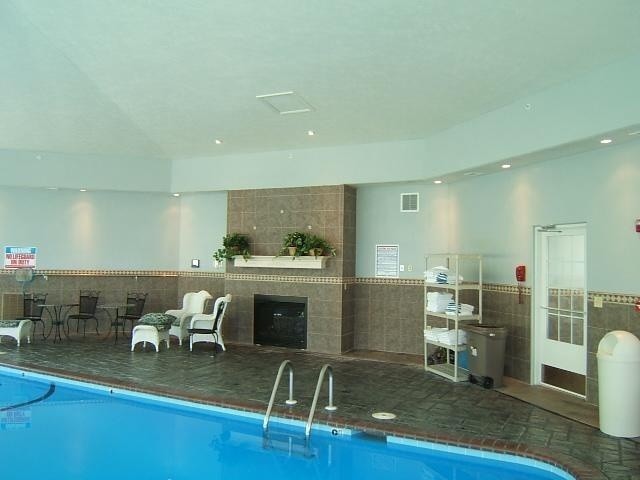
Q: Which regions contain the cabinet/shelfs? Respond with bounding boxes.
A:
[422,253,483,383]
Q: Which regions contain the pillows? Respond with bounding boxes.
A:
[138,313,176,325]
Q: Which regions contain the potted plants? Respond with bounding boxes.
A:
[275,232,337,261]
[212,231,251,263]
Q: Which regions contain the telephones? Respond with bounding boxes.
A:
[516,266,525,281]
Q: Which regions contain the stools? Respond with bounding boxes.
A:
[130,325,170,352]
[0,318,33,347]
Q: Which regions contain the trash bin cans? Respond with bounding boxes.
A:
[596,330,639,438]
[466,324,508,388]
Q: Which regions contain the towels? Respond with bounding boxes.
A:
[423,266,475,347]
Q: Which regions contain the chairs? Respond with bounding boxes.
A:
[16,291,48,341]
[67,289,100,338]
[187,293,233,352]
[164,289,214,346]
[115,292,148,337]
